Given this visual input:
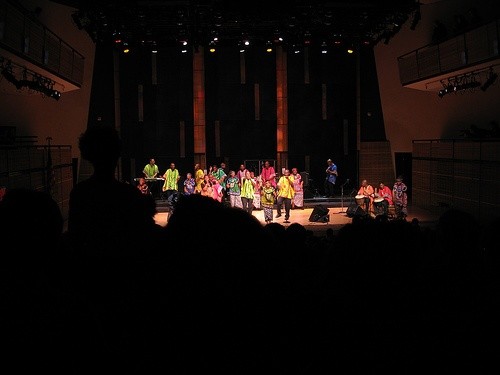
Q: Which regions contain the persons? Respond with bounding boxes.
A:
[357,179,376,218]
[261,160,276,189]
[162,163,181,206]
[143,158,159,178]
[261,180,277,224]
[226,171,243,209]
[183,163,261,209]
[275,169,295,220]
[393,176,407,217]
[378,181,393,205]
[290,167,304,209]
[241,172,257,214]
[323,158,337,198]
[137,178,150,197]
[0,122,500,375]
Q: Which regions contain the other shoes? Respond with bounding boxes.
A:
[275,214,281,218]
[285,215,289,220]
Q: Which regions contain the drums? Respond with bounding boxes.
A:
[355,194,366,210]
[373,197,385,216]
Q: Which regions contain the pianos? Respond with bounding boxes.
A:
[134,177,165,200]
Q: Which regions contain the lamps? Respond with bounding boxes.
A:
[69,0,422,62]
[438,67,498,98]
[0,56,61,101]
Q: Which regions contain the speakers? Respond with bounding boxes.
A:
[308,205,330,222]
